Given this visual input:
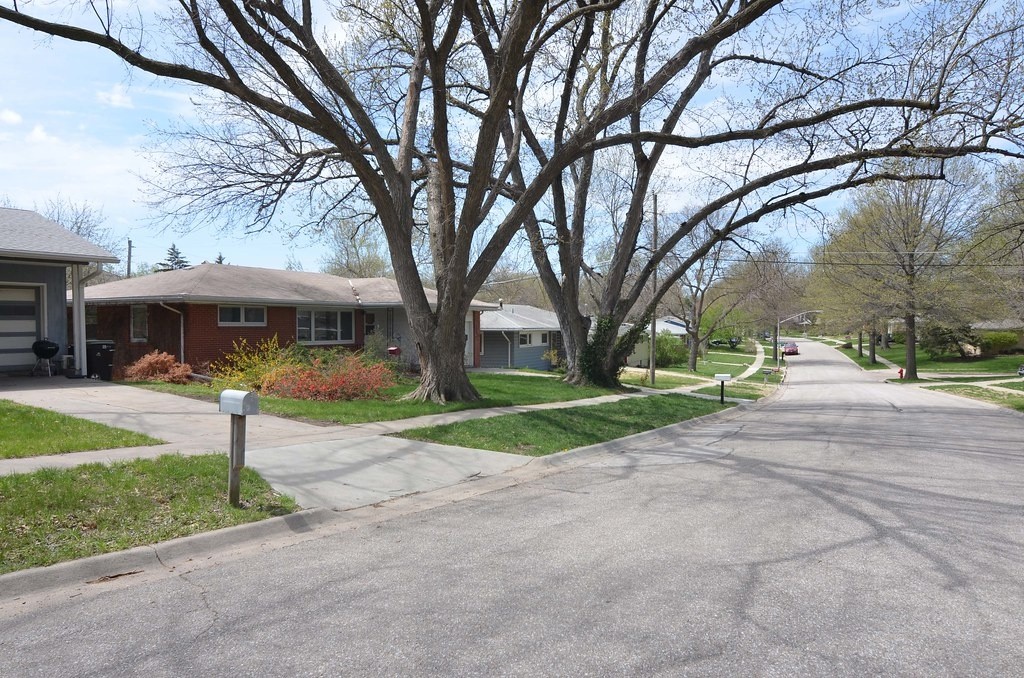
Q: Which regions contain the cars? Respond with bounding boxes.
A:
[877,333,896,345]
[711,337,740,346]
[761,331,770,338]
[784,341,798,355]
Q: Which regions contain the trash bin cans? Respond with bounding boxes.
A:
[85,339,117,382]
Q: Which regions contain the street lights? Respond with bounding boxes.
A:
[777,309,824,371]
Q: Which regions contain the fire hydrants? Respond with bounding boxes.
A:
[898,368,904,380]
